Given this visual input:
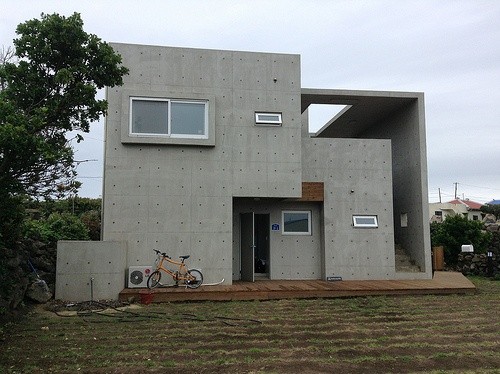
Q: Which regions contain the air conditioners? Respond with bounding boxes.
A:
[127,265,153,288]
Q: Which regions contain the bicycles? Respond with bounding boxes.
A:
[147,248,203,289]
